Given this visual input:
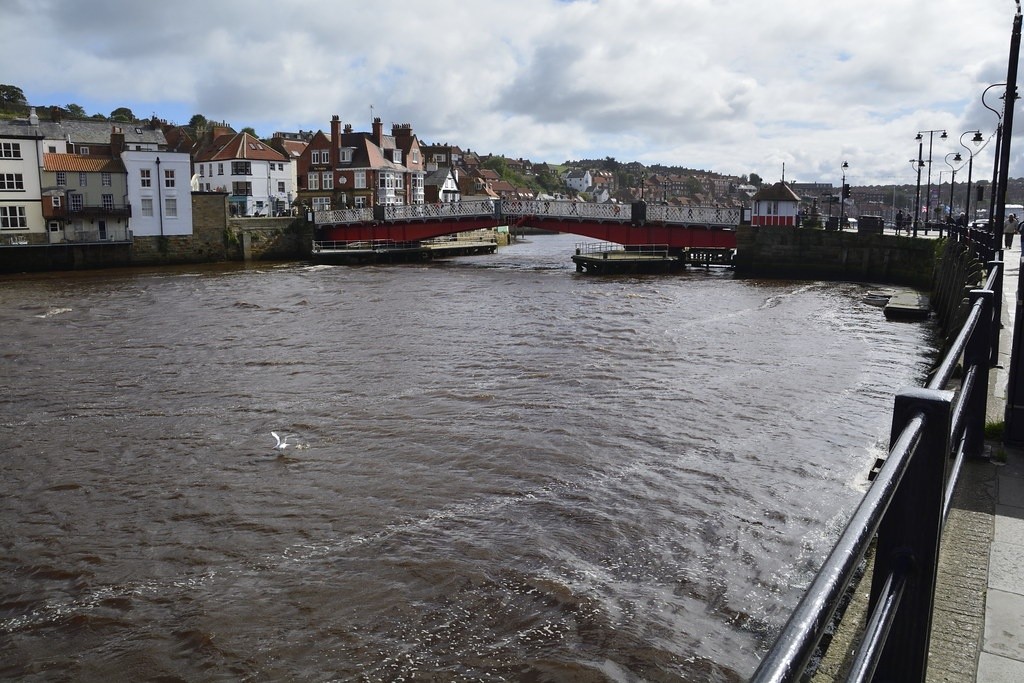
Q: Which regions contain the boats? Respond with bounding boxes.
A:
[311,239,434,265]
[571,242,678,273]
[862,296,889,306]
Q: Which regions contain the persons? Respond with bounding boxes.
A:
[386,196,495,218]
[1003,213,1018,250]
[650,203,740,224]
[502,197,631,218]
[894,209,904,235]
[945,215,956,238]
[957,214,967,241]
[906,213,913,236]
[843,211,849,228]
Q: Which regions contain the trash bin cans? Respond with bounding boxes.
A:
[829,217,839,231]
[858,215,885,234]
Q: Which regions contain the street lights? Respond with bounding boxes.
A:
[822,190,832,216]
[840,161,849,231]
[960,130,985,227]
[945,153,963,238]
[909,143,933,237]
[639,171,645,201]
[915,130,949,236]
[982,84,1022,232]
[662,174,669,206]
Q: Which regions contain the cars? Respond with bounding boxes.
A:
[481,197,498,208]
[838,218,858,226]
[380,203,397,213]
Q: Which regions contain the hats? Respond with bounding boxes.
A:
[899,210,902,212]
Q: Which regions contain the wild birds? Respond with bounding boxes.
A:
[270,431,298,451]
